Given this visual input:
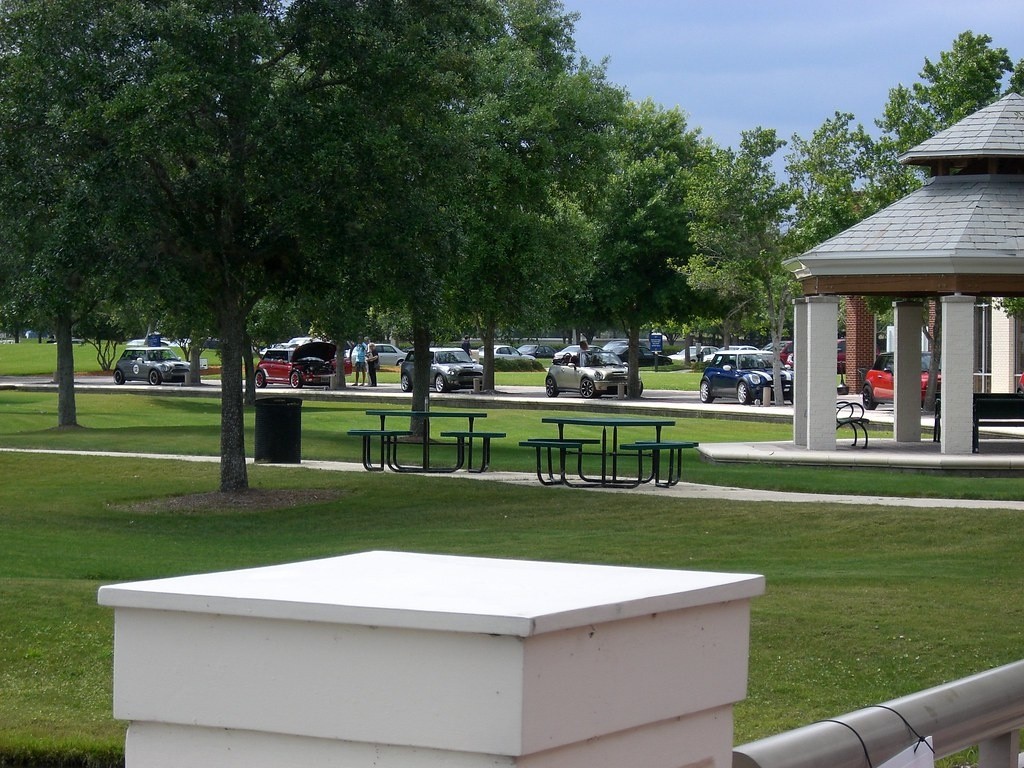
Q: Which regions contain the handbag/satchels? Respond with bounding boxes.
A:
[374,362,380,370]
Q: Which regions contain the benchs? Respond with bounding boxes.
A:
[836,401,869,448]
[619,441,699,488]
[441,431,506,475]
[519,438,601,488]
[347,430,414,474]
[934,391,1024,453]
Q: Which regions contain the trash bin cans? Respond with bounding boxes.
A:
[252,397,303,464]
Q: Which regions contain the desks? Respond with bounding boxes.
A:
[366,410,487,473]
[542,417,675,489]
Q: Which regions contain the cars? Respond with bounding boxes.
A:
[669,345,719,362]
[699,350,794,405]
[343,343,407,366]
[553,345,604,365]
[761,340,794,365]
[866,352,942,410]
[704,346,759,362]
[517,344,558,358]
[610,345,673,366]
[254,340,336,388]
[113,347,190,385]
[545,350,643,399]
[478,344,535,366]
[602,339,647,352]
[259,337,332,358]
[837,339,881,374]
[128,334,219,349]
[400,347,484,393]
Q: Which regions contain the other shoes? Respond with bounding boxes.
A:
[370,384,376,387]
[352,384,358,386]
[359,384,363,386]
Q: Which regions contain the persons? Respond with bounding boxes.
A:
[462,335,473,359]
[352,337,381,388]
[577,340,590,367]
